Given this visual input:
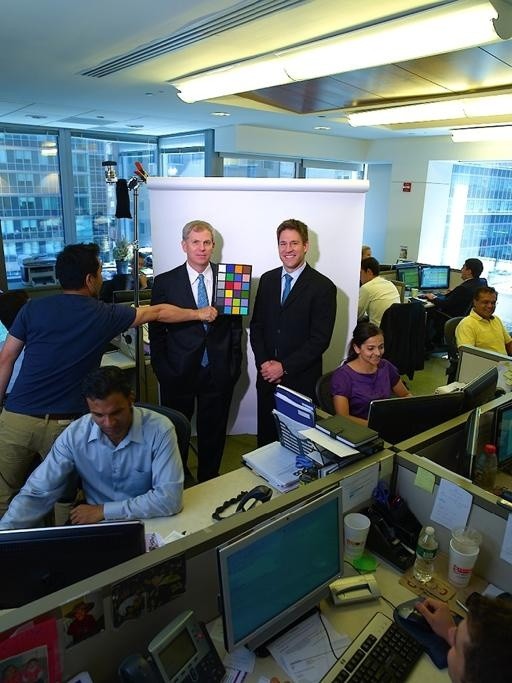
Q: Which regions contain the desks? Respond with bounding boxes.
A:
[173,529,512,681]
[94,349,135,378]
[404,287,450,308]
[119,442,305,552]
[476,461,512,503]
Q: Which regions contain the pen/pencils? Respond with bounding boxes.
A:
[456,600,469,612]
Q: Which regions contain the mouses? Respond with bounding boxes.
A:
[502,491,512,503]
[398,607,433,628]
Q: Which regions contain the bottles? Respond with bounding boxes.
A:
[405,284,410,297]
[411,526,440,585]
[473,444,498,491]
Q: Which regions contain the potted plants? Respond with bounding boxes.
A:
[111,235,130,274]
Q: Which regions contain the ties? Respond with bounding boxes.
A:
[197,274,210,367]
[282,275,293,306]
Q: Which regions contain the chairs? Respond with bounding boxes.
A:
[442,314,469,385]
[131,402,191,472]
[376,299,430,377]
[434,302,472,322]
[314,368,336,413]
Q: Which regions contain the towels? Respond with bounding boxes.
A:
[114,178,132,219]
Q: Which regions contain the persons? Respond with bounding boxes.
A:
[332,321,415,428]
[357,256,401,331]
[0,242,220,530]
[361,245,372,259]
[0,365,185,530]
[62,601,99,642]
[251,218,337,449]
[21,658,44,682]
[147,220,242,490]
[1,664,22,682]
[417,255,488,357]
[269,592,510,682]
[454,287,511,362]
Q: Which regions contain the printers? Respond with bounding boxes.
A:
[20,253,57,287]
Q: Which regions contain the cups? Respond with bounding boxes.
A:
[344,509,373,569]
[412,288,418,297]
[447,525,482,590]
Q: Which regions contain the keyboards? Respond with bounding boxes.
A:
[319,612,425,683]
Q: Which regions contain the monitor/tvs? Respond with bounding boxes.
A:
[491,399,511,476]
[464,408,480,479]
[0,520,147,610]
[379,262,450,294]
[215,485,344,658]
[368,391,464,446]
[461,365,498,413]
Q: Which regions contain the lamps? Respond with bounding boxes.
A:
[163,0,512,107]
[345,83,512,146]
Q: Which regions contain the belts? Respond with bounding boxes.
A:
[32,413,81,419]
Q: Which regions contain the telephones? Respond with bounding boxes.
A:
[118,607,226,683]
[236,485,273,512]
